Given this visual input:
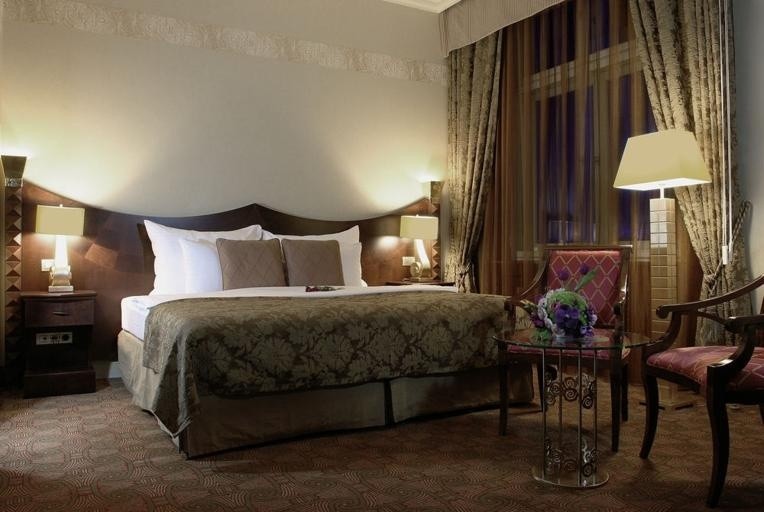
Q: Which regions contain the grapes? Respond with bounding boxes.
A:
[384,280,453,286]
[17,291,99,399]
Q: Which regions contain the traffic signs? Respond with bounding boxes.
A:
[35,333,72,344]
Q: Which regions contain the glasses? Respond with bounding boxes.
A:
[35,205,85,292]
[1,154,28,393]
[422,179,446,282]
[399,215,439,282]
[612,129,716,410]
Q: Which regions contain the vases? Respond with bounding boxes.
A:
[521,268,607,345]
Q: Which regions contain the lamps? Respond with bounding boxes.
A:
[497,244,633,452]
[640,275,764,512]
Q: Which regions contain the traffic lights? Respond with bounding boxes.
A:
[281,239,347,287]
[218,237,286,289]
[182,240,224,293]
[340,244,367,291]
[261,226,366,288]
[142,219,262,295]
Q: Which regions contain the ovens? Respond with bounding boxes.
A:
[116,220,536,458]
